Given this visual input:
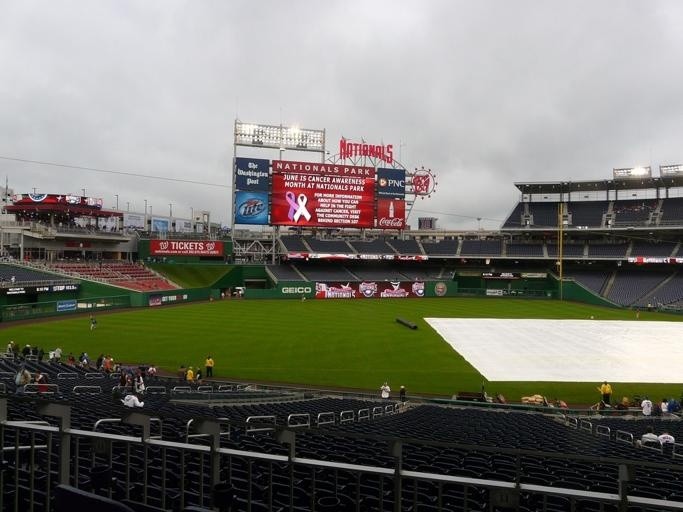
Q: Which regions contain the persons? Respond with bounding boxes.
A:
[590,379,683,447]
[7,340,215,408]
[400,385,406,402]
[380,381,391,399]
[89,316,97,331]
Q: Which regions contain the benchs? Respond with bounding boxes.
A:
[0,263,183,290]
